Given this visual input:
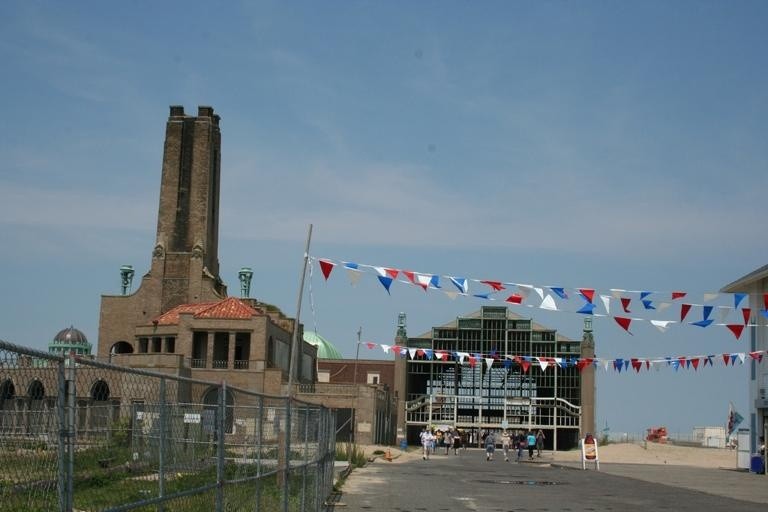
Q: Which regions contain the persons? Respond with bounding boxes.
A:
[484,431,497,460]
[526,431,537,461]
[442,431,452,455]
[453,432,460,455]
[422,428,432,460]
[515,430,524,461]
[432,432,437,453]
[419,428,426,447]
[536,429,546,457]
[501,431,512,461]
[461,428,466,450]
[436,428,442,453]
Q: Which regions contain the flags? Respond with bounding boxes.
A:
[725,401,744,443]
